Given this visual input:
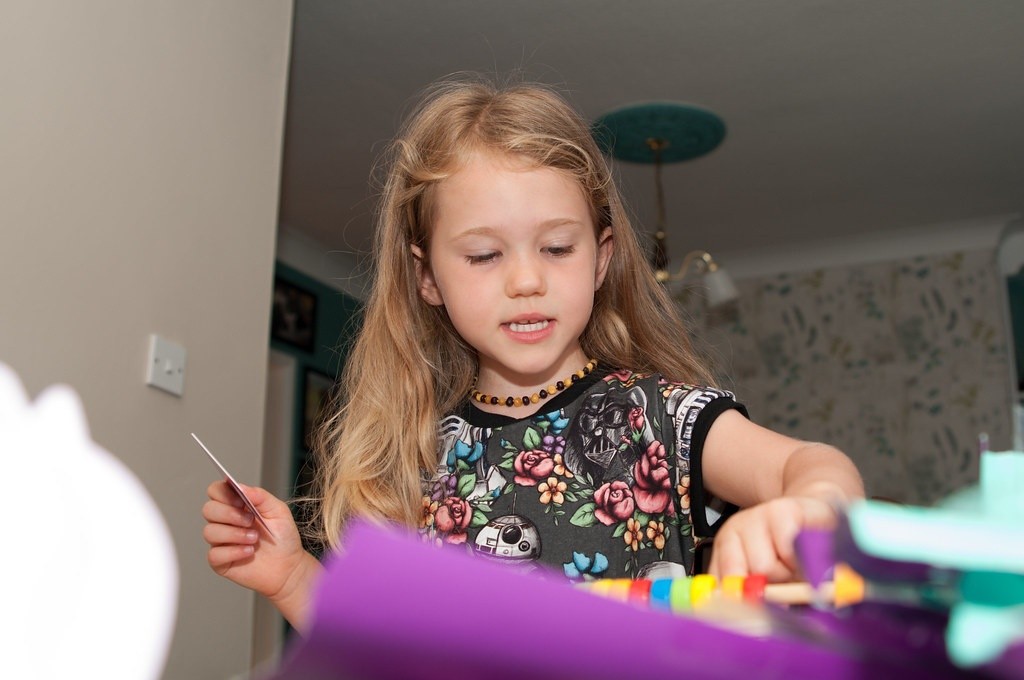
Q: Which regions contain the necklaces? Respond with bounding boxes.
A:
[470,359,597,406]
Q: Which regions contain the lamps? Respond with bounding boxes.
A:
[590,102,744,319]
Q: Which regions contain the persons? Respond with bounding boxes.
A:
[204,84,864,641]
